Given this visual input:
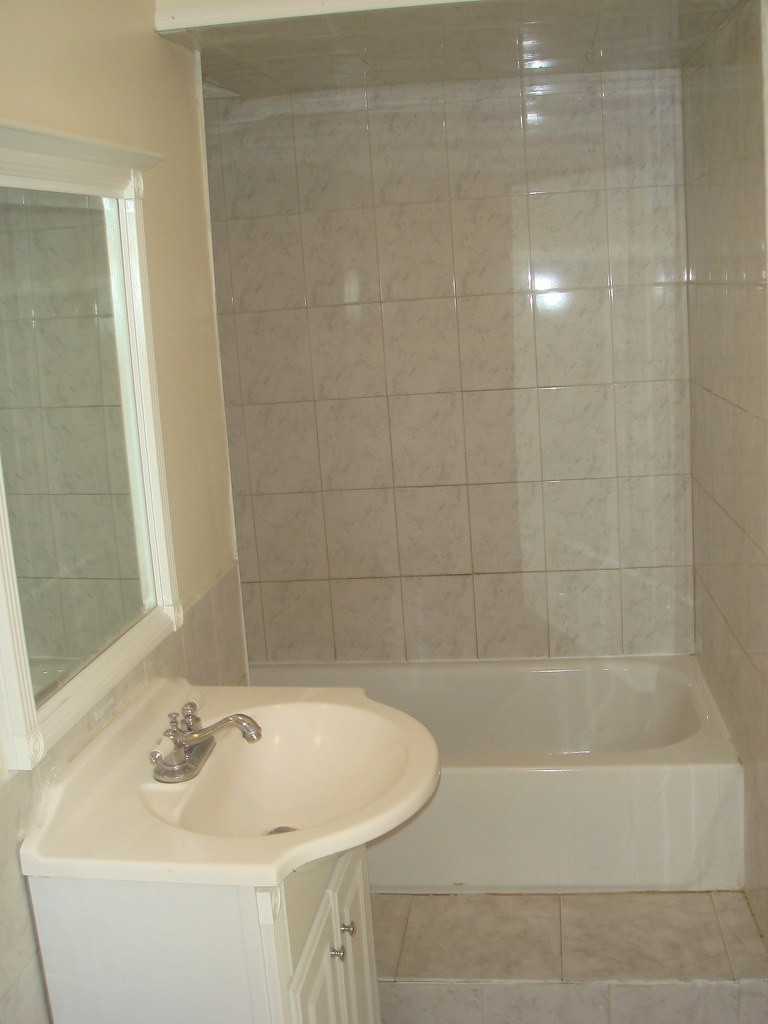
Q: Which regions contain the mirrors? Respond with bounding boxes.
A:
[0,119,188,771]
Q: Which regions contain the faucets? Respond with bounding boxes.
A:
[165,713,263,765]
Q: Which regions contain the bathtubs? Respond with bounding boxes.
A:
[250,655,746,892]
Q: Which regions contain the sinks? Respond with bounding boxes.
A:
[176,704,407,839]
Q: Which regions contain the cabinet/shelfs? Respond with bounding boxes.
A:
[26,840,381,1023]
